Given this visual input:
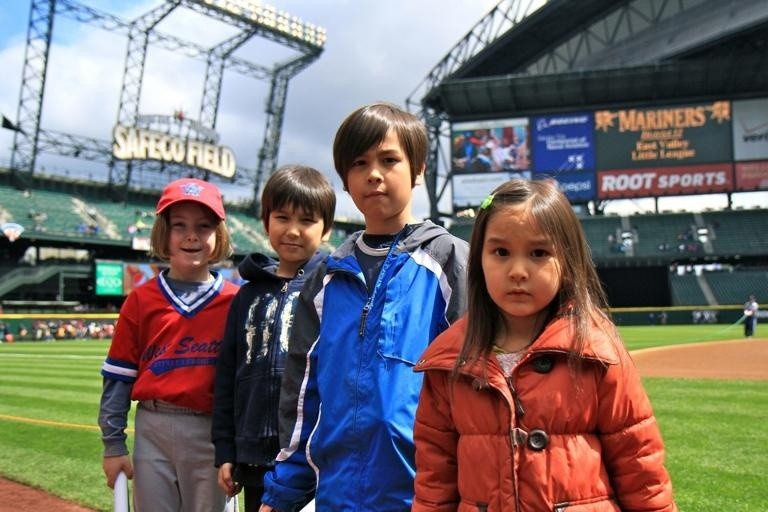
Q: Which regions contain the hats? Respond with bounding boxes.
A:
[156,178,225,220]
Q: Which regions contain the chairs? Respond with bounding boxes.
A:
[451,209,768,264]
[667,265,767,307]
[0,308,120,341]
[0,186,348,258]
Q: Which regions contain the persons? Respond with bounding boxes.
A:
[0,319,118,342]
[98,176,242,512]
[452,135,530,173]
[257,103,470,511]
[209,163,337,511]
[743,294,759,339]
[407,177,681,512]
